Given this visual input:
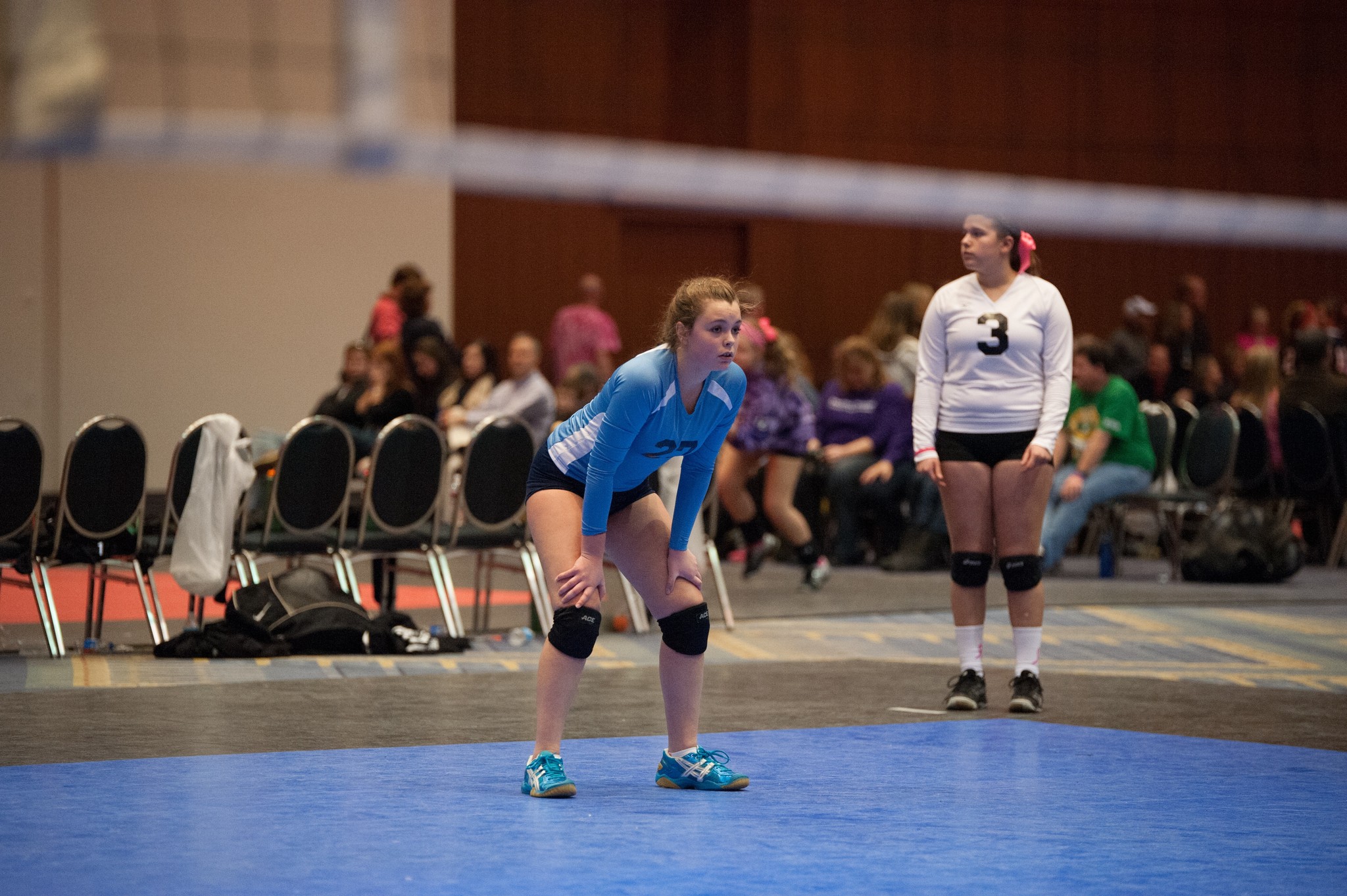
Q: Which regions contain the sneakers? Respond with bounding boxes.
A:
[654,745,750,791]
[522,753,577,799]
[1008,670,1044,713]
[942,668,988,709]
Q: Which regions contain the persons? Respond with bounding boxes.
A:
[310,263,1347,593]
[912,215,1071,714]
[520,277,749,799]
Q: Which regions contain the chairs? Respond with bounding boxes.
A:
[0,398,1347,659]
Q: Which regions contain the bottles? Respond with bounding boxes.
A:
[84,637,117,651]
[507,627,536,646]
[426,625,448,637]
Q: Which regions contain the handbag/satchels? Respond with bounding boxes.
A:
[224,566,370,642]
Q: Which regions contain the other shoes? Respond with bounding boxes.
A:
[742,535,781,575]
[800,555,834,593]
[880,522,937,569]
